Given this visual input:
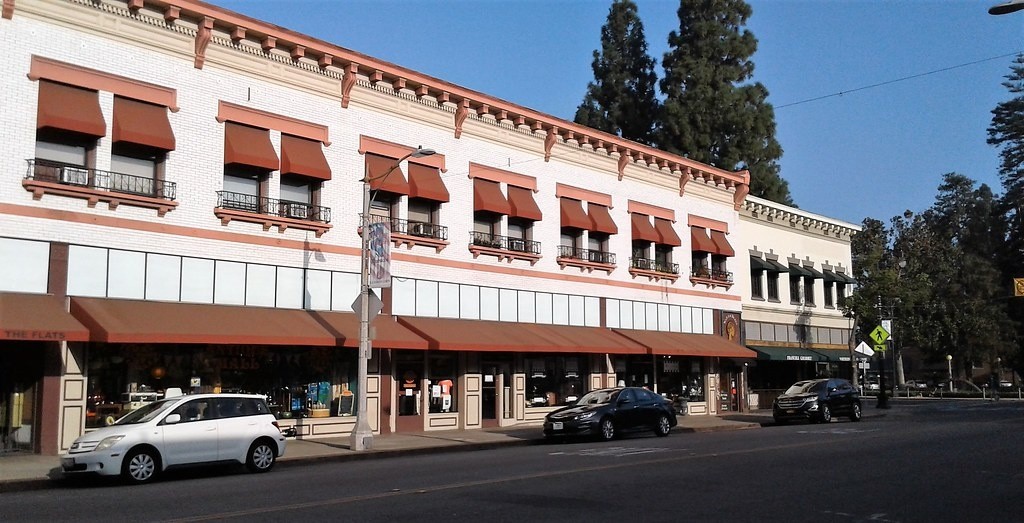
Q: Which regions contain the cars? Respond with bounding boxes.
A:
[61,393,287,486]
[865,380,880,390]
[543,386,678,443]
[999,380,1013,388]
[904,379,929,391]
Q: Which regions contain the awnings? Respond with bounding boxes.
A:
[408,161,450,205]
[752,346,827,365]
[752,254,856,285]
[630,212,659,245]
[0,288,89,342]
[397,317,646,354]
[692,226,717,256]
[69,294,335,346]
[611,327,755,361]
[472,180,510,215]
[38,80,107,138]
[810,347,874,365]
[655,218,683,248]
[115,94,176,152]
[281,130,333,180]
[309,308,429,351]
[590,203,618,238]
[712,230,736,258]
[508,184,543,221]
[366,154,410,197]
[562,196,595,233]
[223,119,279,174]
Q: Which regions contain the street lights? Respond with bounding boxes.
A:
[946,355,953,392]
[350,144,436,451]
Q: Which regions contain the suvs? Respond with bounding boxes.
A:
[772,378,862,426]
